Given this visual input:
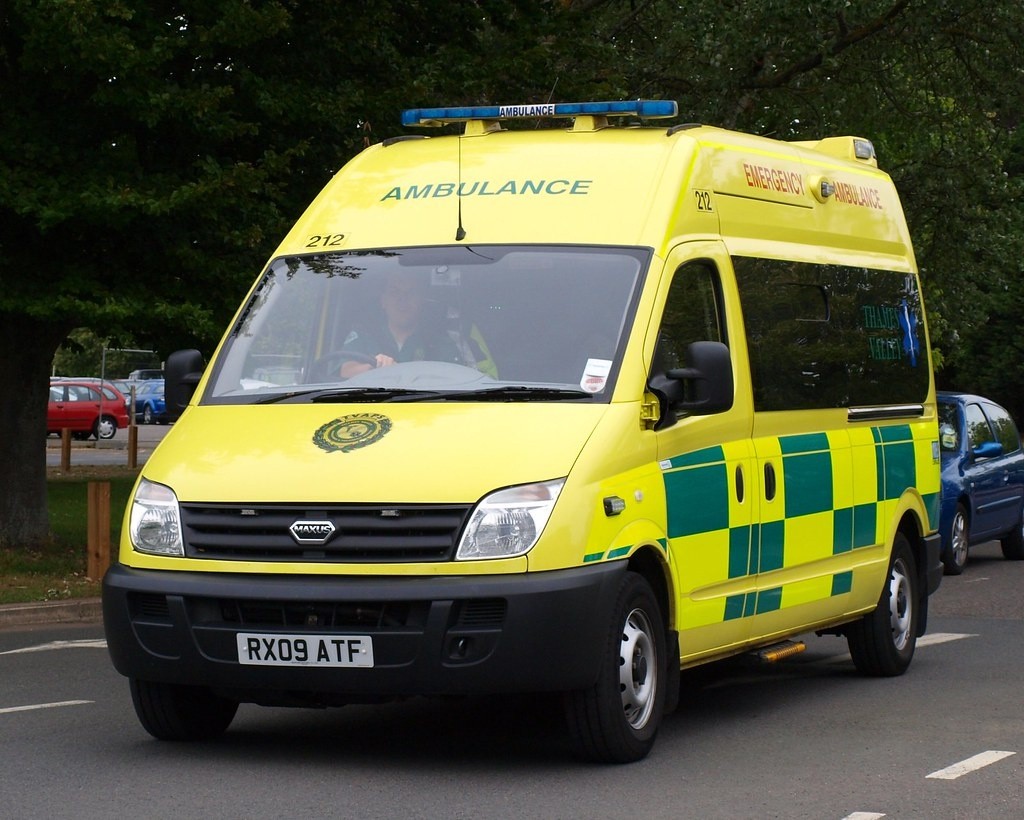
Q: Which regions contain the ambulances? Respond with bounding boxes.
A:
[98,100,947,766]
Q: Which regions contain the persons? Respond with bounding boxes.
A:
[339,261,500,392]
[551,323,627,392]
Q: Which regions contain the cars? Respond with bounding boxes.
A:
[49,369,177,423]
[933,391,1024,577]
[45,381,130,440]
